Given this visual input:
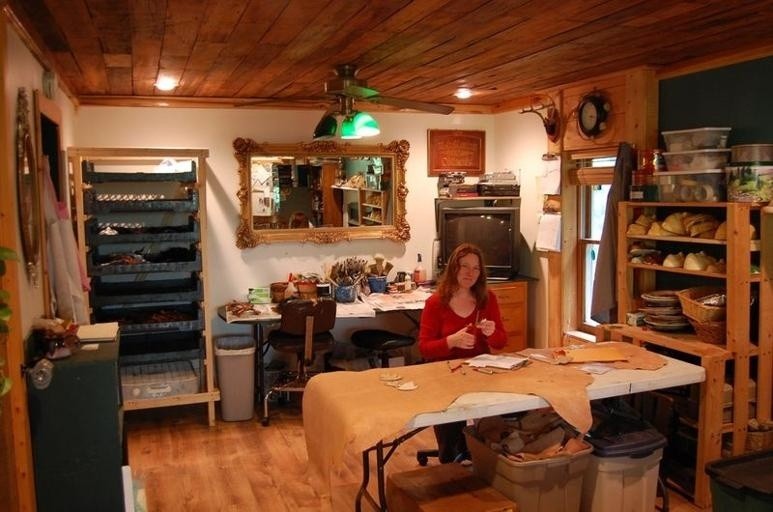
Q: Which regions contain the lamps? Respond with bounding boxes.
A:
[312,109,379,138]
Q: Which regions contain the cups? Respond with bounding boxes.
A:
[367,277,388,294]
[269,282,286,302]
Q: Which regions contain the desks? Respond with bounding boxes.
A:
[214,282,433,406]
[301,339,709,512]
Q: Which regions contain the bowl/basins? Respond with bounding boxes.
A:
[722,165,773,202]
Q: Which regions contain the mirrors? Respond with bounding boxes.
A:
[231,135,409,247]
[14,85,42,286]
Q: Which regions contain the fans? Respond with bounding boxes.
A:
[234,61,453,116]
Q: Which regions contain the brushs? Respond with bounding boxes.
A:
[369,257,394,277]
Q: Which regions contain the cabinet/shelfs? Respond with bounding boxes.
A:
[306,162,344,227]
[594,195,772,512]
[488,280,528,351]
[26,323,145,512]
[66,138,220,431]
[356,184,388,227]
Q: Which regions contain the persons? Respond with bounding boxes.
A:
[288,212,311,228]
[416,243,509,468]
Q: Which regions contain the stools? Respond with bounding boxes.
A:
[350,328,415,370]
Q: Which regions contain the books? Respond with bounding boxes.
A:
[75,321,118,343]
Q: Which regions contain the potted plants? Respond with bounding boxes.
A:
[323,256,367,302]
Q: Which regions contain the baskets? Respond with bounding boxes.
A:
[675,283,755,323]
[688,319,726,345]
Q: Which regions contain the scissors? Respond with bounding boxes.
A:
[466,311,488,345]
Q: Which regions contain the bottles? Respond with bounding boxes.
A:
[397,252,426,293]
[284,282,299,299]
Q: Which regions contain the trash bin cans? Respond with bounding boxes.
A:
[213,334,257,422]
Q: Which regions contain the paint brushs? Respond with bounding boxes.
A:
[330,256,368,287]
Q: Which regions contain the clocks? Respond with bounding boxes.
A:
[577,89,610,140]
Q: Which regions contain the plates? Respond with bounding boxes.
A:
[637,290,689,332]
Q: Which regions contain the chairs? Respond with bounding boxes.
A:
[260,298,336,426]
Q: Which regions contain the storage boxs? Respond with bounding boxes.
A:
[652,169,724,203]
[387,464,518,512]
[660,126,731,151]
[661,148,731,171]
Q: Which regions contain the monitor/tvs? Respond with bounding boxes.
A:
[441,207,520,281]
